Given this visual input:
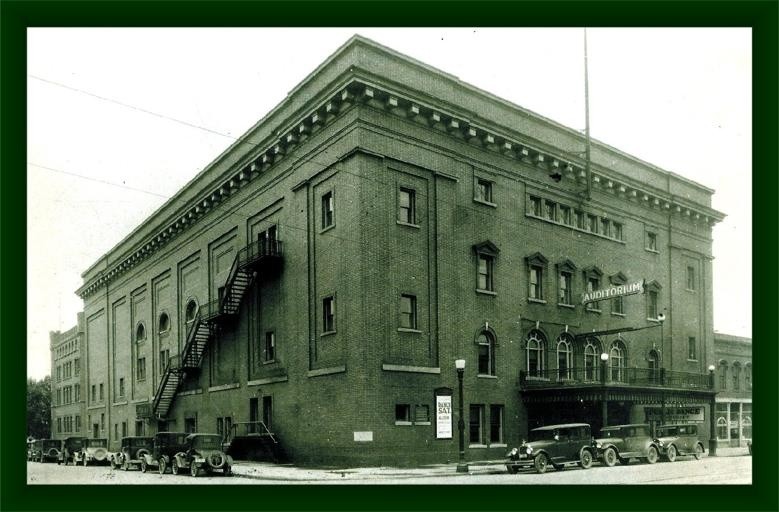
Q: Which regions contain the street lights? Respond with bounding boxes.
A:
[708,363,718,457]
[453,357,470,472]
[601,351,609,425]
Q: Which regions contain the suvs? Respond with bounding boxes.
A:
[27,431,235,477]
[505,422,706,475]
